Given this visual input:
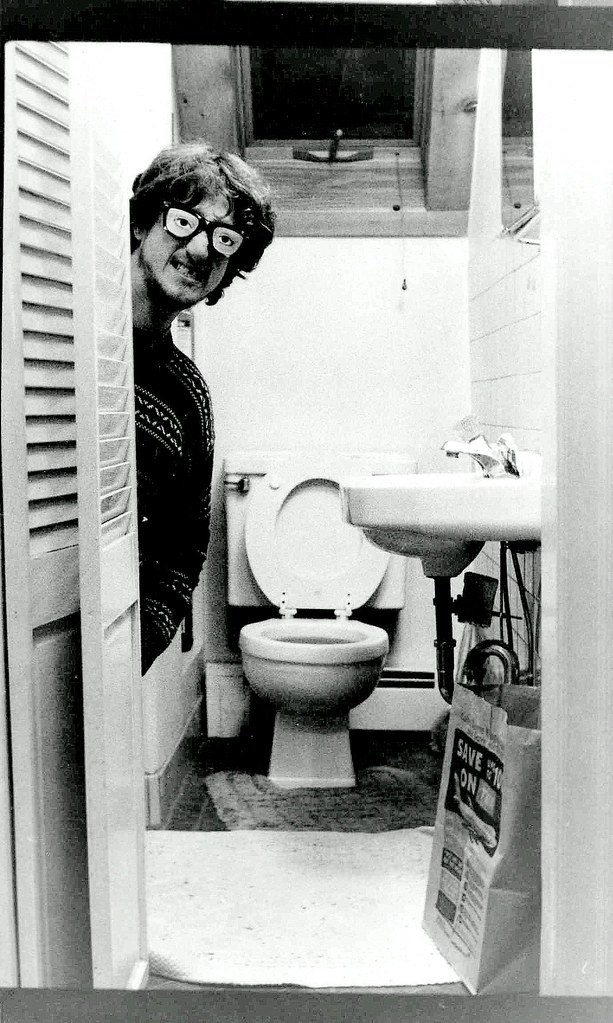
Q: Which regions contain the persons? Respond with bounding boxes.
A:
[126,143,275,675]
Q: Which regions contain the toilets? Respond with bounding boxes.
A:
[222,449,419,789]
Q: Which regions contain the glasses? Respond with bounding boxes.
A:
[156,194,255,263]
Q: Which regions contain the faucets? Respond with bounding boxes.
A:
[438,413,523,479]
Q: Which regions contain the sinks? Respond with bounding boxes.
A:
[346,469,542,578]
